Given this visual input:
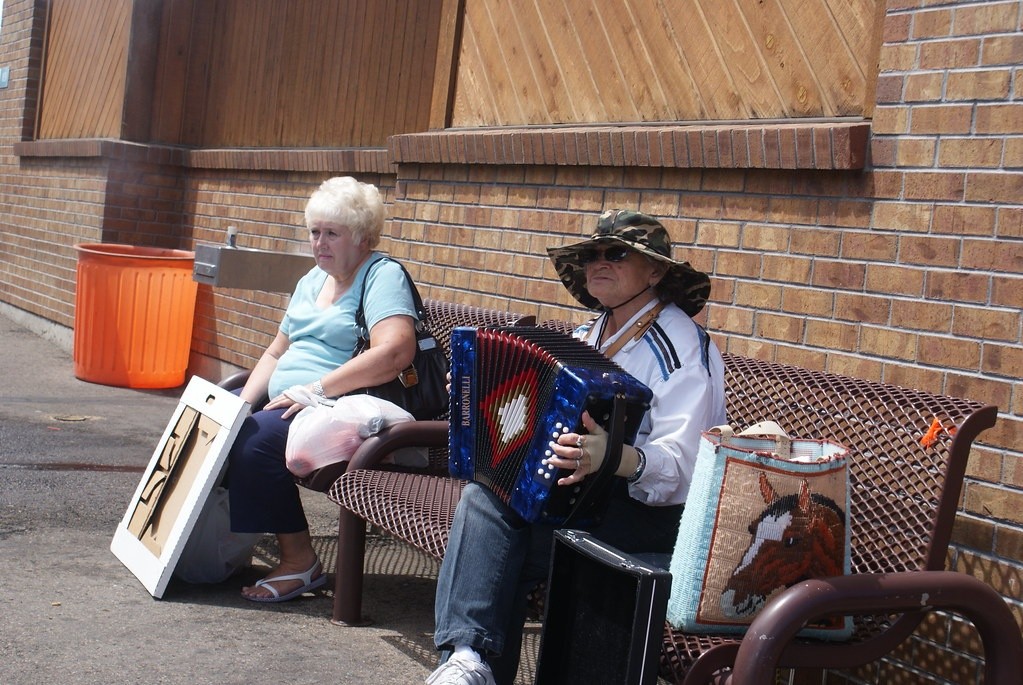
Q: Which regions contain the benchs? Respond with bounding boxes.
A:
[211,294,534,626]
[326,322,1023,684]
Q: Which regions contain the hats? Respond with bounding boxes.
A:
[545,208,710,318]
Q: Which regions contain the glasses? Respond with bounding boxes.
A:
[576,247,638,262]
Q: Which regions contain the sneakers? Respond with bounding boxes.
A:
[427,658,497,685]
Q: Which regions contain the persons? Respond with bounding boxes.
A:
[428,207,726,685]
[219,177,419,601]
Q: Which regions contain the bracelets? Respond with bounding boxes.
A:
[314,376,327,400]
[628,451,645,480]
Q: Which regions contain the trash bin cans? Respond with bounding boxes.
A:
[71,240,198,390]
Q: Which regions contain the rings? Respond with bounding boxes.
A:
[575,434,583,447]
[575,459,579,469]
[578,447,584,459]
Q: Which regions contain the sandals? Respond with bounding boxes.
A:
[242,556,328,602]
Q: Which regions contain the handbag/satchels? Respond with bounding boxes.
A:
[171,487,262,586]
[668,419,859,641]
[283,386,430,476]
[343,258,452,420]
